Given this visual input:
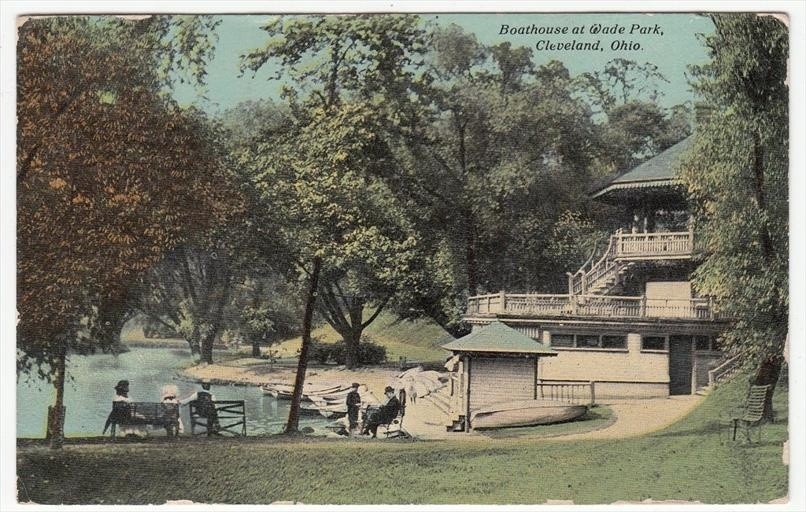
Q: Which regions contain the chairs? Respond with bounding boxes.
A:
[733,385,771,446]
[362,389,407,440]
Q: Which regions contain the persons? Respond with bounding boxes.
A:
[345,382,361,438]
[161,383,184,434]
[113,379,145,438]
[399,377,406,405]
[359,385,400,439]
[408,377,418,404]
[180,378,221,437]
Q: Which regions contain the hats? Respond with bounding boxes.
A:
[351,383,360,388]
[384,386,395,394]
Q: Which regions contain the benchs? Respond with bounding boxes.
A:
[107,401,246,440]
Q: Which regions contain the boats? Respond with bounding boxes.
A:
[257,381,404,440]
[467,398,590,431]
